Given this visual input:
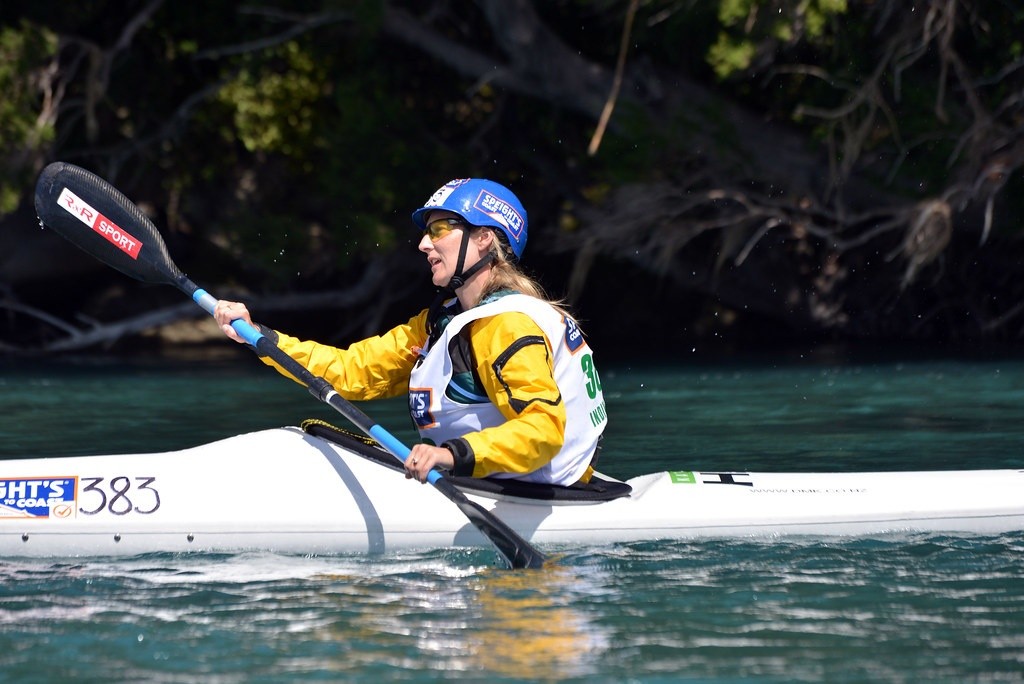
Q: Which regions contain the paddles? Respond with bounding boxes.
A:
[31,161,545,572]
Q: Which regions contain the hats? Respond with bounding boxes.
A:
[412,178,528,260]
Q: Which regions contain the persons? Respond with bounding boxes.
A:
[214,179,611,486]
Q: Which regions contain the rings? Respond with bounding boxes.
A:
[411,457,417,464]
[228,305,233,310]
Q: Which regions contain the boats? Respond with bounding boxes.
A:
[0,418,1024,557]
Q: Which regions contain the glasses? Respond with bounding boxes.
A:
[423,218,465,243]
